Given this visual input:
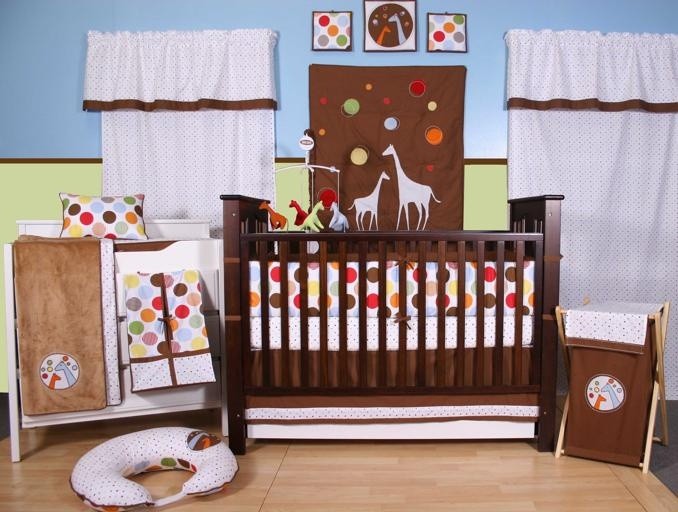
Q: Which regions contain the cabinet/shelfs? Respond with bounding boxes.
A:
[2,220,225,462]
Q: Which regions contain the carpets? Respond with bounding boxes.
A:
[647,398,678,498]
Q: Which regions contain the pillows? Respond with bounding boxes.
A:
[68,426,240,511]
[59,191,147,241]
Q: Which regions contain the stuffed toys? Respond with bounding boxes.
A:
[329,202,348,232]
[300,201,326,232]
[289,199,308,226]
[257,199,288,231]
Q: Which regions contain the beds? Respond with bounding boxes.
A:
[219,194,566,456]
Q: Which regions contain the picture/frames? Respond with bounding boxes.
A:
[363,0,418,53]
[312,10,353,52]
[427,12,467,53]
